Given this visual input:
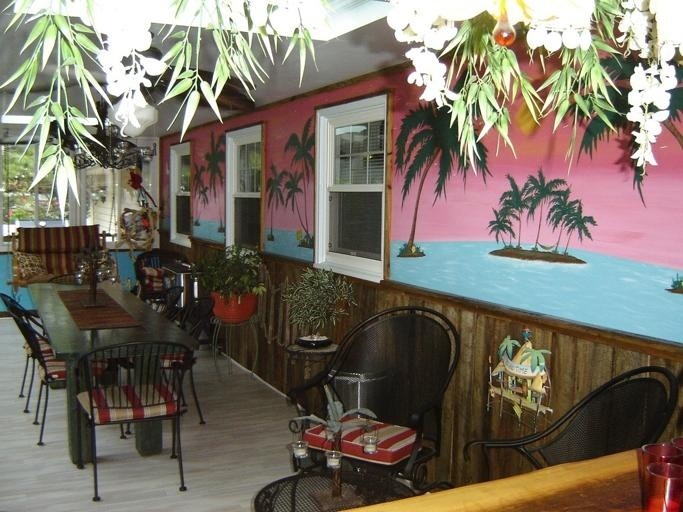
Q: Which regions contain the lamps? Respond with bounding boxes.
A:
[61,101,155,171]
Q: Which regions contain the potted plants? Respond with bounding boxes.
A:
[189,243,266,324]
[280,267,359,349]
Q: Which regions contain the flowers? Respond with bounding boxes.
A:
[0,0,682,210]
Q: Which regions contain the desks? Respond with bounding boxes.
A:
[252,471,416,512]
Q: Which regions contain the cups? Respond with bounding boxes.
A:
[325,451,343,470]
[292,440,309,459]
[363,432,378,455]
[641,436,683,512]
